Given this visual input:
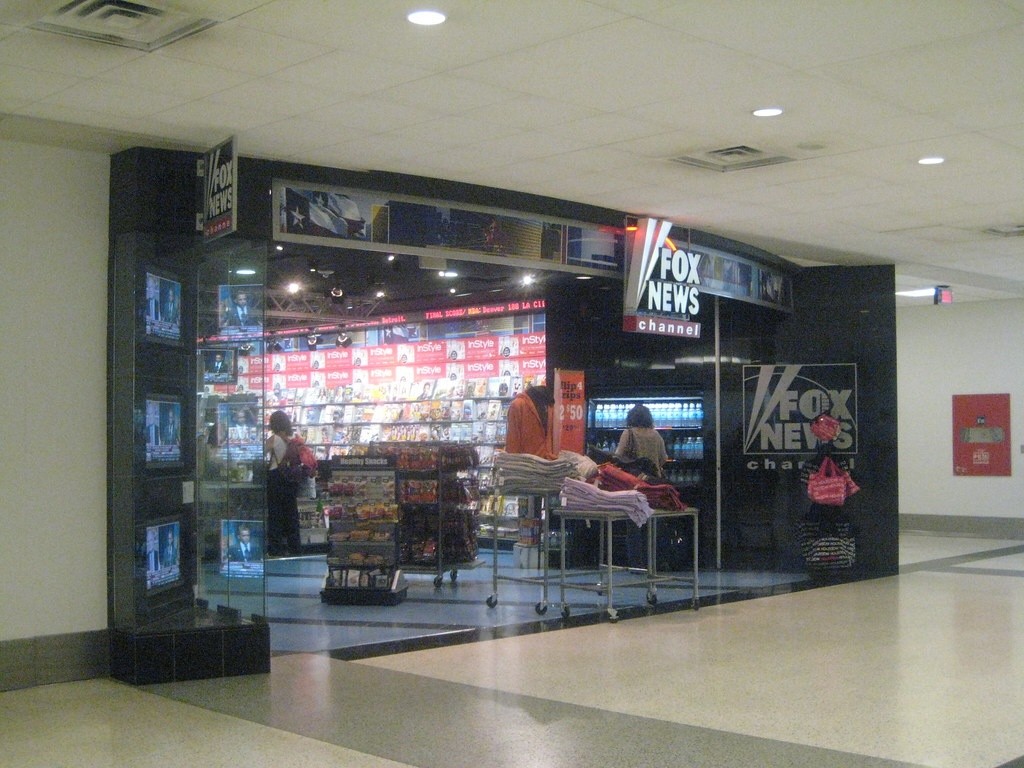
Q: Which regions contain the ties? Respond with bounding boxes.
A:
[150,548,172,573]
[150,425,172,446]
[244,545,251,562]
[216,362,220,368]
[239,306,249,326]
[149,299,172,319]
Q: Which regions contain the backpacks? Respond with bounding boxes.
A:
[272,432,318,490]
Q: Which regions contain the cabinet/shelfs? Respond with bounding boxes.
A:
[212,440,484,607]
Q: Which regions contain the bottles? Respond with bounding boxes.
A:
[666,467,699,485]
[596,436,704,457]
[594,402,705,427]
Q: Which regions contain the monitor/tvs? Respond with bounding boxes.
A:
[221,520,264,579]
[219,285,263,343]
[217,402,264,462]
[199,349,238,386]
[146,272,182,341]
[144,396,184,467]
[136,515,183,597]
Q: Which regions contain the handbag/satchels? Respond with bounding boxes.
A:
[798,410,864,572]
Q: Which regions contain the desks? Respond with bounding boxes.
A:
[486,486,699,624]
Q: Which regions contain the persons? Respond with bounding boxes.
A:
[318,389,326,399]
[414,403,419,412]
[146,402,177,445]
[613,406,669,574]
[229,524,257,562]
[146,530,177,572]
[453,380,464,399]
[145,277,177,323]
[232,408,250,427]
[417,383,430,399]
[219,289,259,326]
[417,432,427,441]
[208,353,228,373]
[266,410,302,558]
[505,385,553,458]
[512,377,521,395]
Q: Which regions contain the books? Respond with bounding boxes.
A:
[267,374,546,516]
[479,523,519,538]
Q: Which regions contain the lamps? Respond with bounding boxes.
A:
[306,333,322,351]
[267,337,283,354]
[238,342,256,357]
[337,329,352,347]
[330,286,345,304]
